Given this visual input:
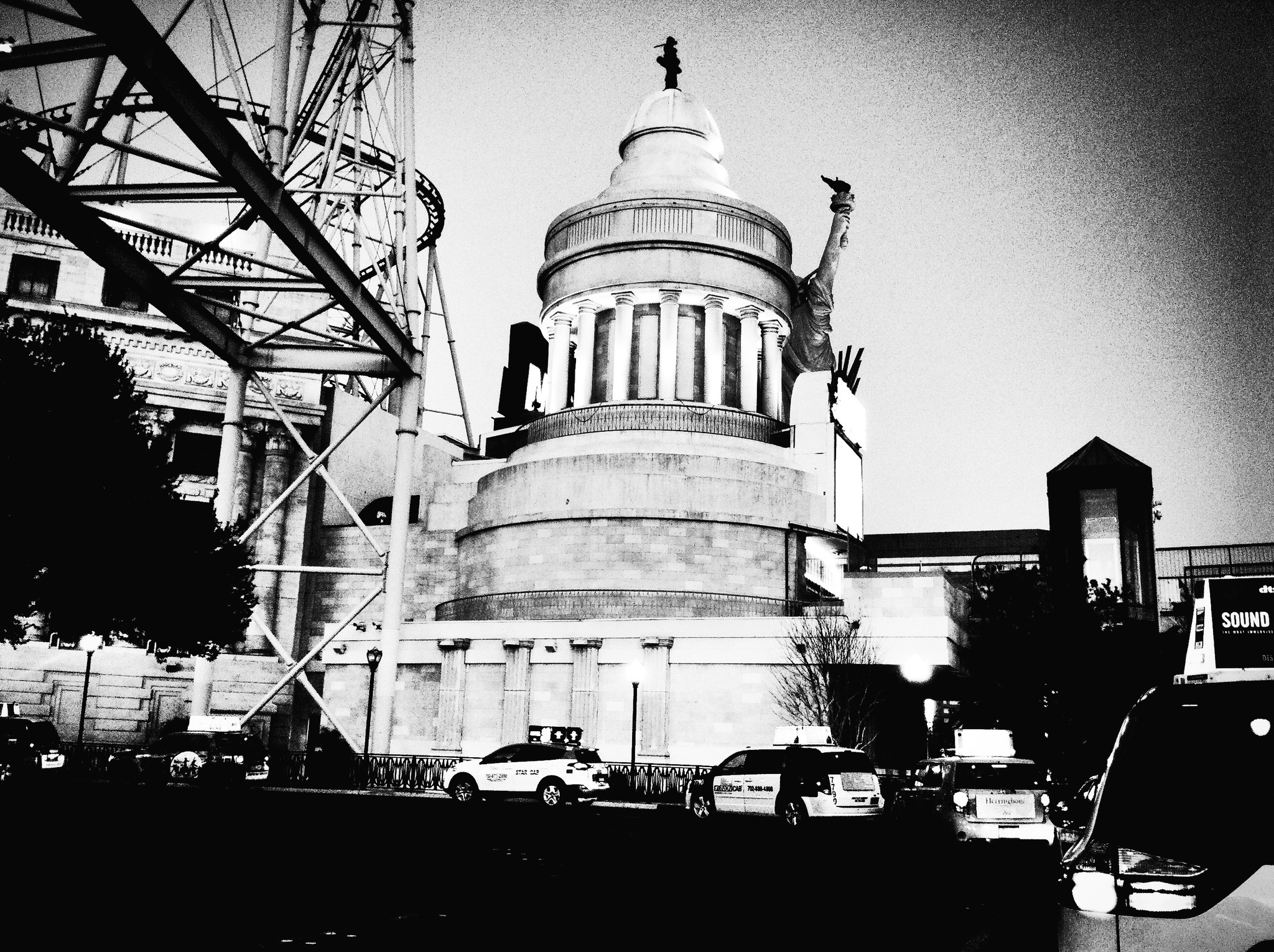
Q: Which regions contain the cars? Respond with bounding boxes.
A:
[444,743,610,807]
[688,743,885,829]
[0,716,66,782]
[892,755,1062,871]
[107,730,273,791]
[1049,668,1274,952]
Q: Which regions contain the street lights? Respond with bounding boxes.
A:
[69,635,102,766]
[358,646,383,788]
[630,664,647,789]
